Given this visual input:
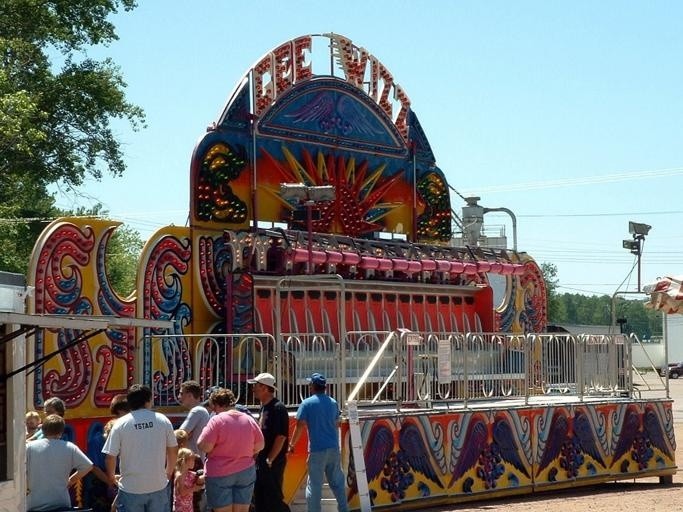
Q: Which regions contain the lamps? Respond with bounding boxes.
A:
[280,181,307,200]
[629,222,648,235]
[307,185,336,202]
[623,239,640,249]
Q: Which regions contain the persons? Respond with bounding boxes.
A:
[287,372,348,511]
[25,372,291,512]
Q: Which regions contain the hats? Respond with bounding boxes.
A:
[246,372,280,392]
[306,372,327,385]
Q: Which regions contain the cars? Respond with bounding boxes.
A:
[660,362,683,379]
